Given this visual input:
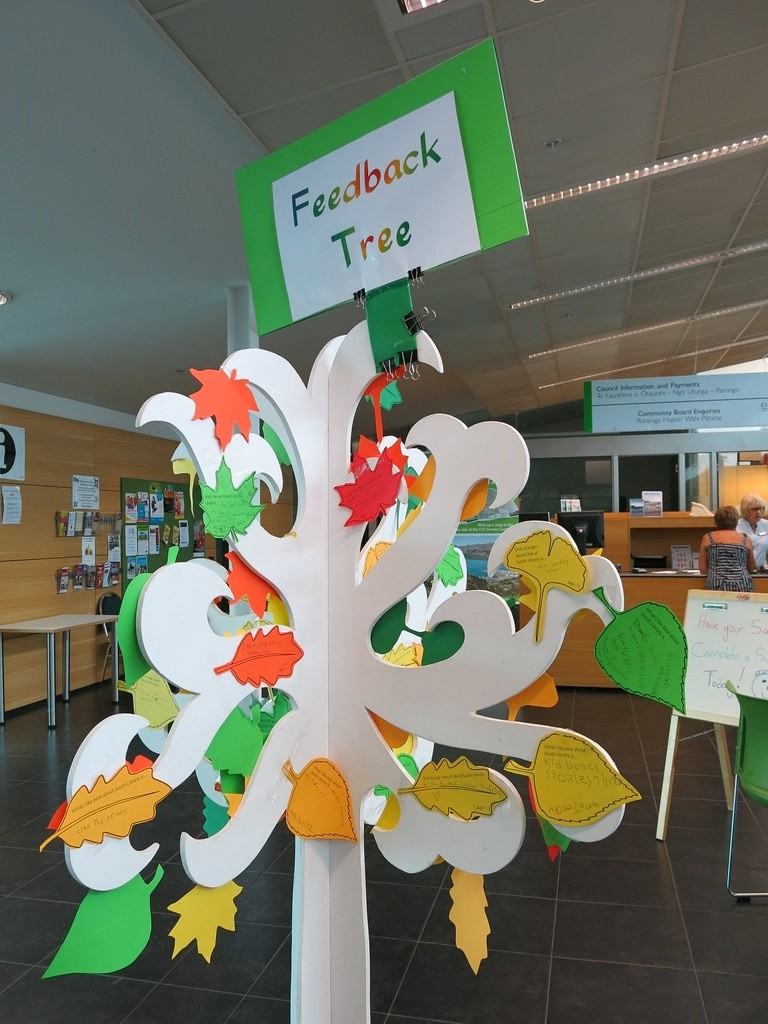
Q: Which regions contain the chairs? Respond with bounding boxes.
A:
[98,591,122,687]
[724,680,768,901]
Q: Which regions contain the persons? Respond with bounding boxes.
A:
[735,494,768,574]
[699,506,756,592]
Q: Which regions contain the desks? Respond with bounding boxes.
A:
[0,613,119,730]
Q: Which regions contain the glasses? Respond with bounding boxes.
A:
[751,508,762,511]
